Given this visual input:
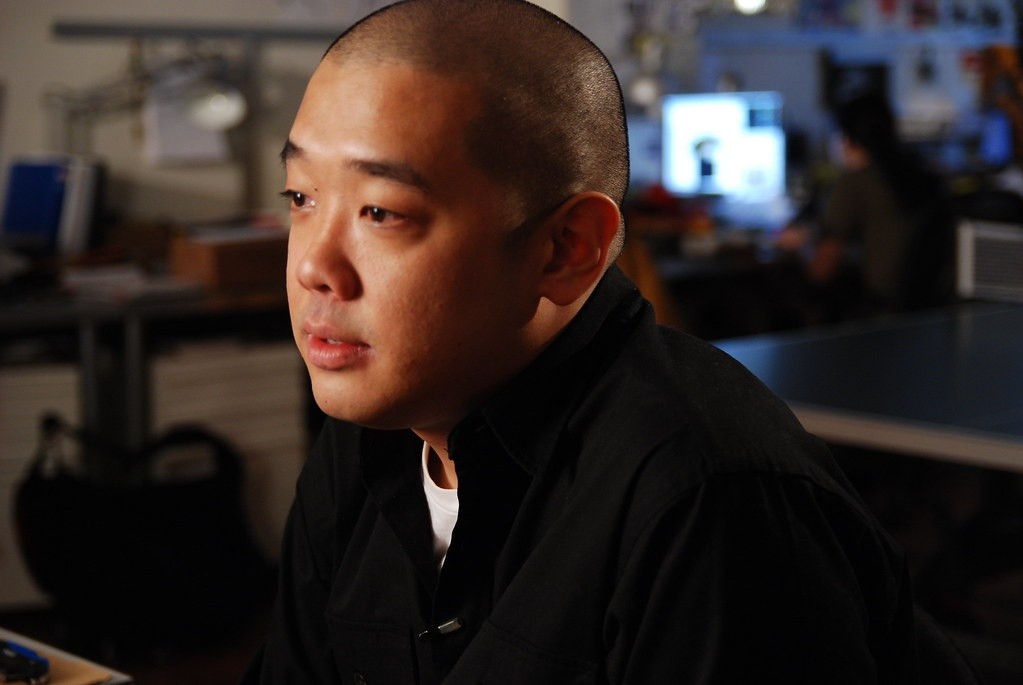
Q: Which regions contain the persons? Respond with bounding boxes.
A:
[244,0,982,685]
[766,67,1023,335]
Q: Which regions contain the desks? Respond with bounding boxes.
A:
[0,283,294,672]
[710,297,1023,639]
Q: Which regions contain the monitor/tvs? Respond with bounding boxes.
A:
[660,90,786,203]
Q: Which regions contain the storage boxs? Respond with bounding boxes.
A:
[167,224,288,288]
